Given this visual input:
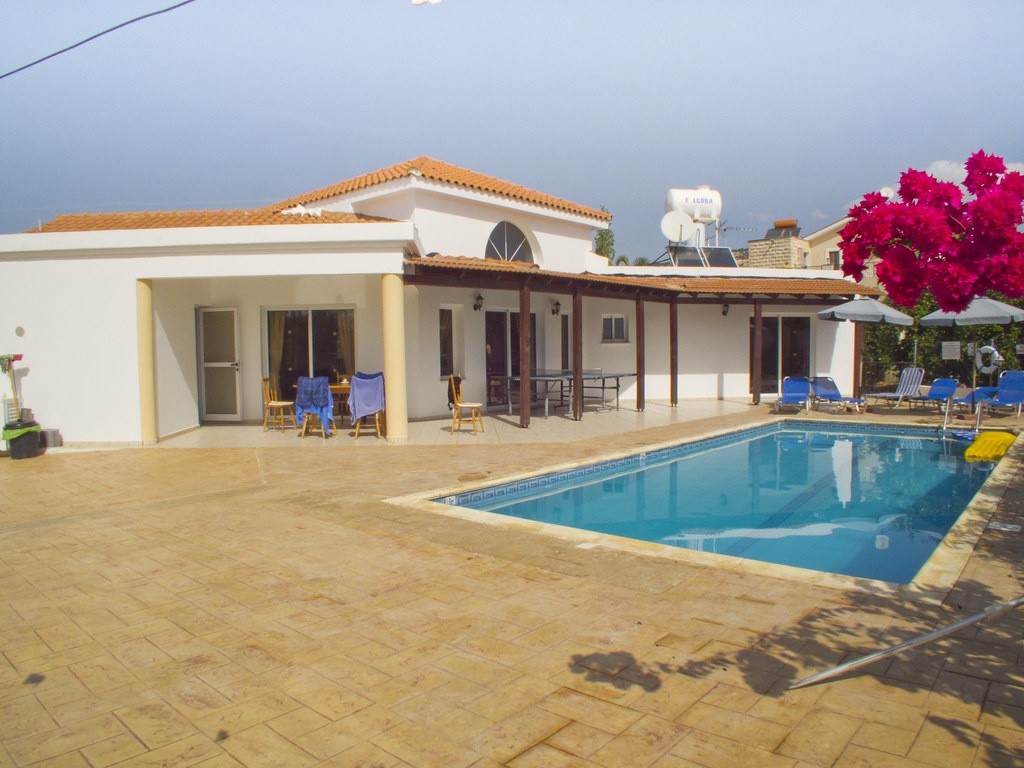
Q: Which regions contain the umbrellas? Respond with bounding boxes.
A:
[919,295,1024,414]
[818,296,914,399]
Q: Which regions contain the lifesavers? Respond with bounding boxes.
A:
[975,346,1000,374]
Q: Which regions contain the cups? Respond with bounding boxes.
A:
[343,379,347,382]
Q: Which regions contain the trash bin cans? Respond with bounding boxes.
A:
[3,420,42,460]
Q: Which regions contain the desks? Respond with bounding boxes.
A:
[490,368,636,419]
[293,384,351,426]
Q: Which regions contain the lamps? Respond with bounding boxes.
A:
[473,294,484,312]
[552,301,561,316]
[722,304,729,314]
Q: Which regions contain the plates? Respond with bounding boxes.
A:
[341,382,349,383]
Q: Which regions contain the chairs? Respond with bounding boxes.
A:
[447,373,484,436]
[296,377,337,437]
[908,379,959,414]
[809,377,865,414]
[861,367,924,411]
[346,376,384,440]
[260,375,297,433]
[774,376,810,415]
[941,387,999,415]
[986,371,1024,418]
